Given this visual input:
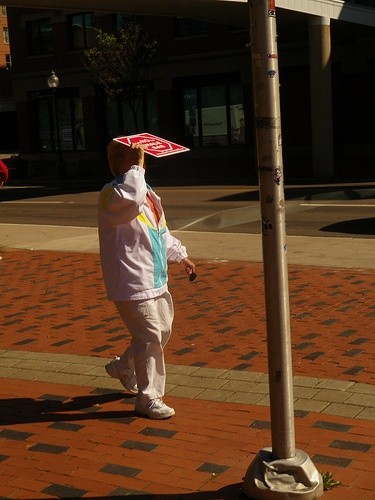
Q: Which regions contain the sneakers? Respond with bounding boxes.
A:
[134,394,176,420]
[105,356,139,396]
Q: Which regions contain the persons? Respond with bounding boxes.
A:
[97,136,195,419]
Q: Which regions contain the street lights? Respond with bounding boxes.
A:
[47,71,62,156]
